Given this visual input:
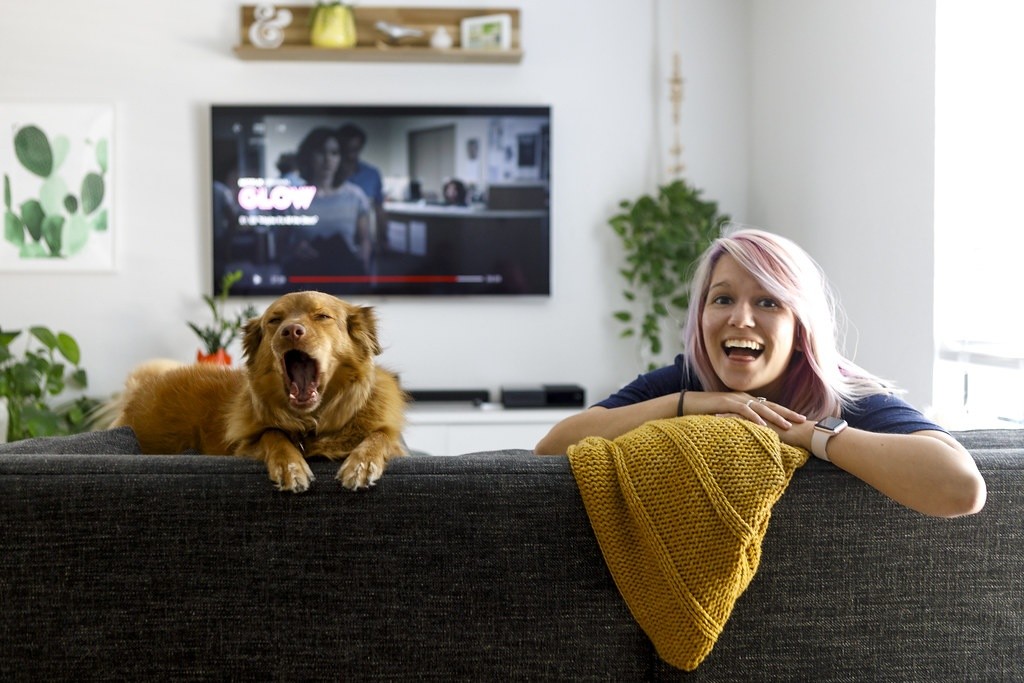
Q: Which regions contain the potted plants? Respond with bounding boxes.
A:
[186,273,256,368]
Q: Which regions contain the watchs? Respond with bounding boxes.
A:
[811,415,848,461]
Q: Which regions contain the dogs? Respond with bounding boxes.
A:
[79,291,414,493]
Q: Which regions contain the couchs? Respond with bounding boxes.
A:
[0,431,1024,683]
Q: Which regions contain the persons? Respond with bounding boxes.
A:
[408,180,476,207]
[213,124,382,294]
[534,229,987,517]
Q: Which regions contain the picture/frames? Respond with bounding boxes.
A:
[460,12,510,49]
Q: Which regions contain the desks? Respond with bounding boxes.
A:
[404,401,587,457]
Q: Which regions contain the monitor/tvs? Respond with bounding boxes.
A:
[210,105,550,298]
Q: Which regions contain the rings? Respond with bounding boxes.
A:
[757,397,766,404]
[747,400,753,407]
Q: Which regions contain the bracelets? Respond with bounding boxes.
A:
[677,389,687,417]
